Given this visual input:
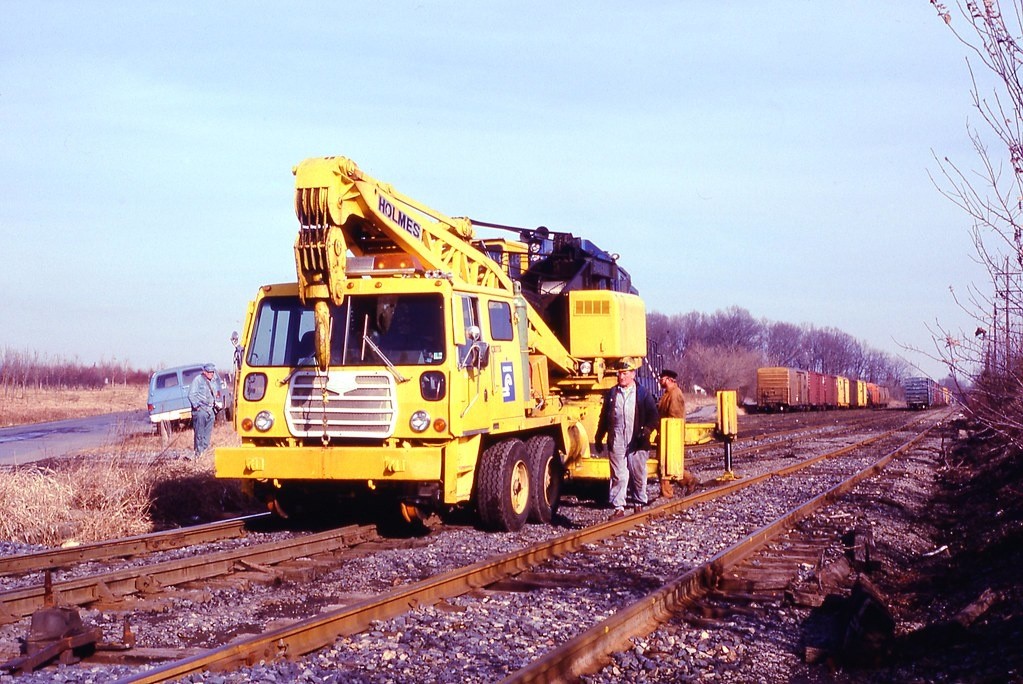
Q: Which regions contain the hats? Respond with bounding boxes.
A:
[659,369,678,379]
[203,364,216,373]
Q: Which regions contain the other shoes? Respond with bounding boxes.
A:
[634,507,643,514]
[609,510,624,520]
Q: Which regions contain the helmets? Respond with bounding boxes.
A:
[617,357,638,370]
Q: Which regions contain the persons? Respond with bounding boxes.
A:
[652,369,701,502]
[187,363,217,462]
[594,356,658,522]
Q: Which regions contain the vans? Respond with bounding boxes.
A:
[148,366,234,433]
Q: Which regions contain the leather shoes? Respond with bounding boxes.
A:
[686,478,700,496]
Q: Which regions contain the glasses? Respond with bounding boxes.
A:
[615,372,633,377]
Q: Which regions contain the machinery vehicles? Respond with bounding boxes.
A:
[209,154,743,537]
